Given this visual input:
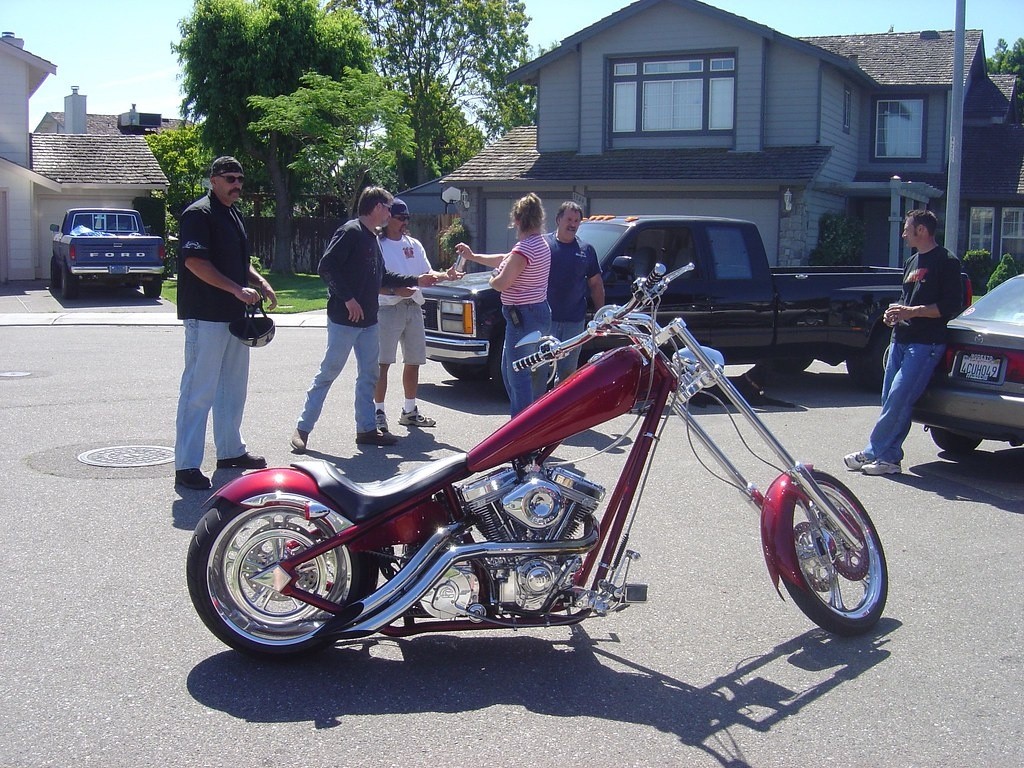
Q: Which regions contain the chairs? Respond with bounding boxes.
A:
[628,246,698,277]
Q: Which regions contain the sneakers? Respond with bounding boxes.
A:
[356,428,397,444]
[217,452,266,469]
[290,428,309,452]
[860,458,902,475]
[175,468,211,490]
[375,409,388,432]
[844,450,875,469]
[399,404,436,426]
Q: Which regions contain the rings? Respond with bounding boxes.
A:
[895,315,898,319]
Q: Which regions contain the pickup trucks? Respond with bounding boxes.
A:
[50,207,166,303]
[416,214,972,403]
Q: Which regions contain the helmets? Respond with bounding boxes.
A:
[229,317,275,347]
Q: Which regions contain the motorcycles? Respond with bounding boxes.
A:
[182,263,892,662]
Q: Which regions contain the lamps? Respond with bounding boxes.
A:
[783,188,792,210]
[462,189,470,209]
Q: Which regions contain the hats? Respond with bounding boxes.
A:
[211,156,243,177]
[390,198,409,214]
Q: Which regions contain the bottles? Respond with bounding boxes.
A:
[456,251,466,272]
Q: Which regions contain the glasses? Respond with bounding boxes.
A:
[220,175,244,184]
[382,203,392,211]
[392,216,409,221]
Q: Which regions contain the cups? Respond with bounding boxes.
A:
[889,304,899,326]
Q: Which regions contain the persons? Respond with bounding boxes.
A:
[290,187,438,452]
[454,192,551,424]
[842,211,962,475]
[374,199,466,430]
[175,157,276,489]
[531,202,604,404]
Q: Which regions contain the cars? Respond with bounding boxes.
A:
[906,272,1024,453]
